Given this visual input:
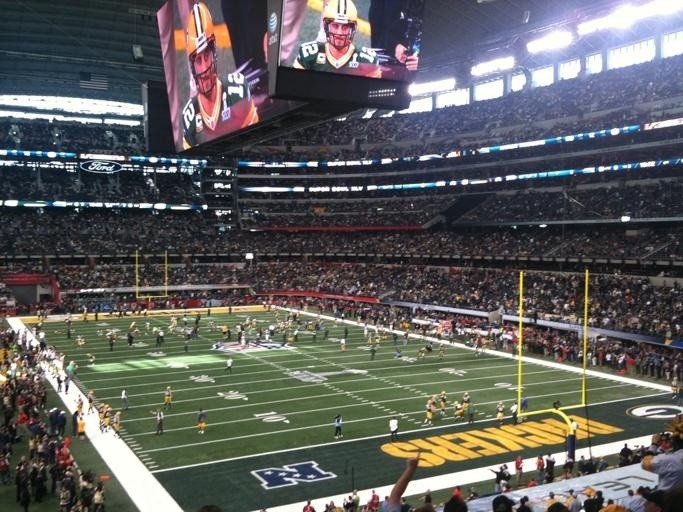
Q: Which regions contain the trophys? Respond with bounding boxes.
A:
[400,10,418,63]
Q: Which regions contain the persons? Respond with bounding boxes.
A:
[390,416,400,441]
[334,414,343,439]
[148,407,166,435]
[162,386,172,406]
[487,414,683,512]
[297,448,479,512]
[195,408,206,436]
[367,0,418,81]
[0,387,131,511]
[293,0,379,79]
[421,394,528,427]
[178,2,258,147]
[1,68,683,391]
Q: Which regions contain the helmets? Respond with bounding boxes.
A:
[322,0,359,48]
[185,2,219,94]
